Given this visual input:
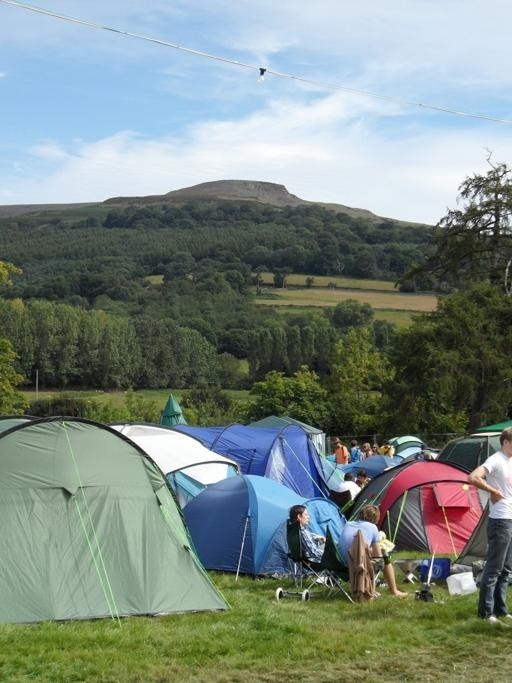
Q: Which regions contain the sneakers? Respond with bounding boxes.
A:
[506,612,512,618]
[489,617,499,623]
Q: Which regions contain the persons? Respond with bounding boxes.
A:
[468,426,512,625]
[338,472,362,501]
[339,503,409,597]
[289,504,338,565]
[332,435,396,464]
[355,469,370,490]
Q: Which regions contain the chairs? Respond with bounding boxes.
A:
[275,518,391,607]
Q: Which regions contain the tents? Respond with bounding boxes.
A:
[387,435,428,457]
[401,450,436,464]
[351,454,400,480]
[172,421,333,501]
[339,458,484,557]
[282,415,327,459]
[181,472,350,579]
[0,414,235,626]
[436,432,504,472]
[245,414,346,491]
[108,423,241,510]
[451,497,490,574]
